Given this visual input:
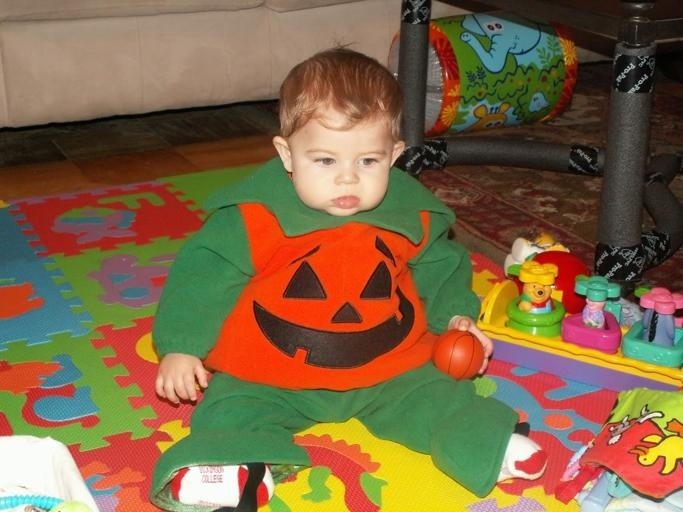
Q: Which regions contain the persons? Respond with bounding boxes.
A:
[147,47,547,511]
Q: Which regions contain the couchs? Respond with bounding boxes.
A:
[1,1,616,165]
